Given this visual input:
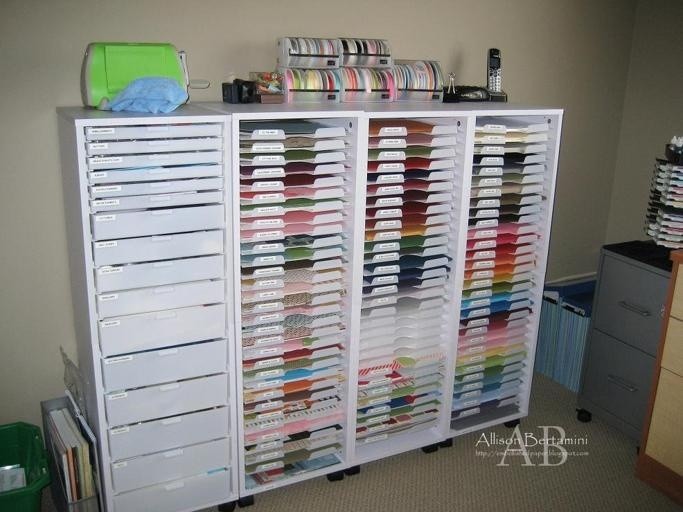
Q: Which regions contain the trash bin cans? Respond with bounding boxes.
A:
[0,422,51,512]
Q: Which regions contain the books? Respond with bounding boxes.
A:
[42,389,104,512]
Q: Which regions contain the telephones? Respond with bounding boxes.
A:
[484,48,507,102]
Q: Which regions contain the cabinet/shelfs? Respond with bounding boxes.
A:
[574,241,673,451]
[56,109,565,512]
